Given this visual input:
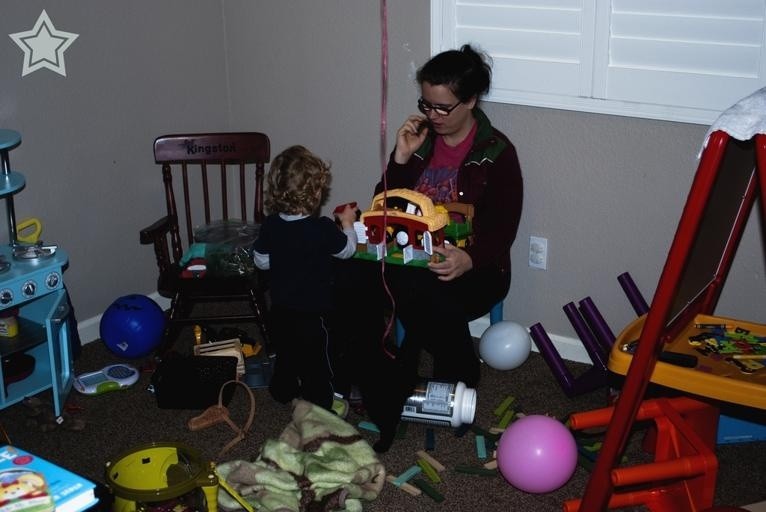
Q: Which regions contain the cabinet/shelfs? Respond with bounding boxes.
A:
[0,131,77,416]
[575,86,766,511]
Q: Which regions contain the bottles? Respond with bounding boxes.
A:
[403,381,476,426]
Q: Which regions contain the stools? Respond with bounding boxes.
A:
[396,300,505,355]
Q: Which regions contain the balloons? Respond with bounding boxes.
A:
[495,414,578,492]
[477,320,531,371]
[98,293,165,357]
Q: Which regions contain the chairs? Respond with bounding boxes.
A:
[528,271,658,400]
[565,396,721,512]
[140,131,275,379]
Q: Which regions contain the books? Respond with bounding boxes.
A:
[0,443,101,512]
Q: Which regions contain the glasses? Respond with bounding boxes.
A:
[417,95,467,117]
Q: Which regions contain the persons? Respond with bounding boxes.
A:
[372,44,523,388]
[250,143,358,399]
